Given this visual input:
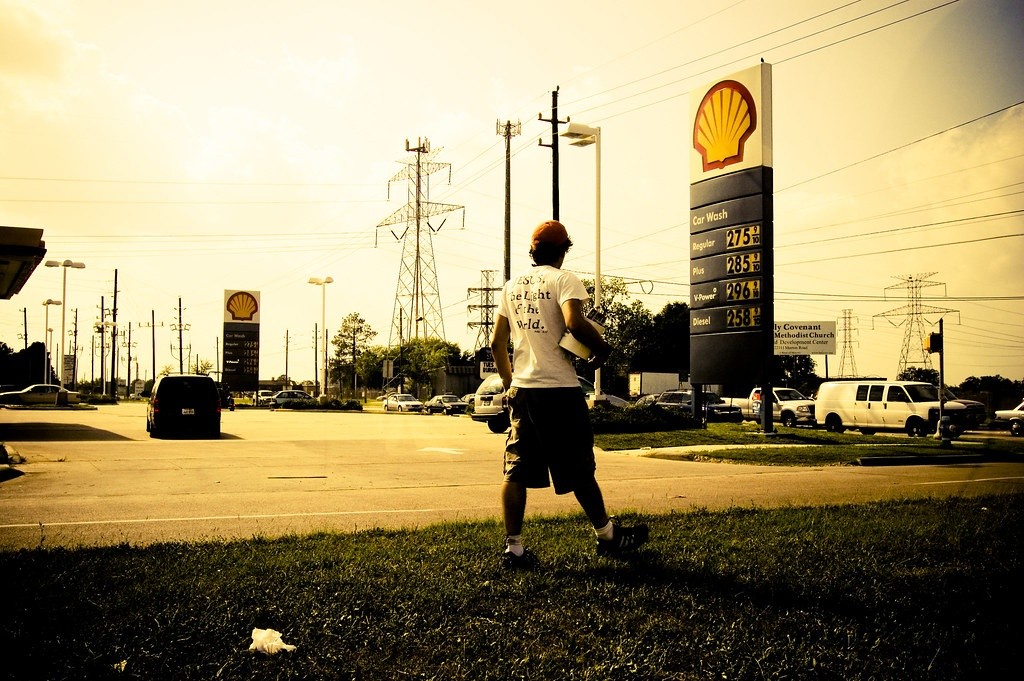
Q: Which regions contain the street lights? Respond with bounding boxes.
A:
[307,276,335,403]
[40,261,87,387]
[562,120,602,394]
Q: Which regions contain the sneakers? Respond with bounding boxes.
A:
[597,516,648,558]
[502,545,540,572]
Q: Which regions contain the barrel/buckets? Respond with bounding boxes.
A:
[56,392,68,406]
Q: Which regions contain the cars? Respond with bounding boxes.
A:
[473,369,630,435]
[995,400,1024,437]
[383,393,478,415]
[254,391,320,404]
[0,384,85,405]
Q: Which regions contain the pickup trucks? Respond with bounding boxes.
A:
[720,387,815,424]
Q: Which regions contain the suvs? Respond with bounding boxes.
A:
[651,391,737,419]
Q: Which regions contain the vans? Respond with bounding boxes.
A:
[145,373,222,440]
[814,381,988,435]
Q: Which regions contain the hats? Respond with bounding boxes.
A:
[531,220,568,245]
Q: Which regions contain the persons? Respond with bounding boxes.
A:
[490,221,651,568]
